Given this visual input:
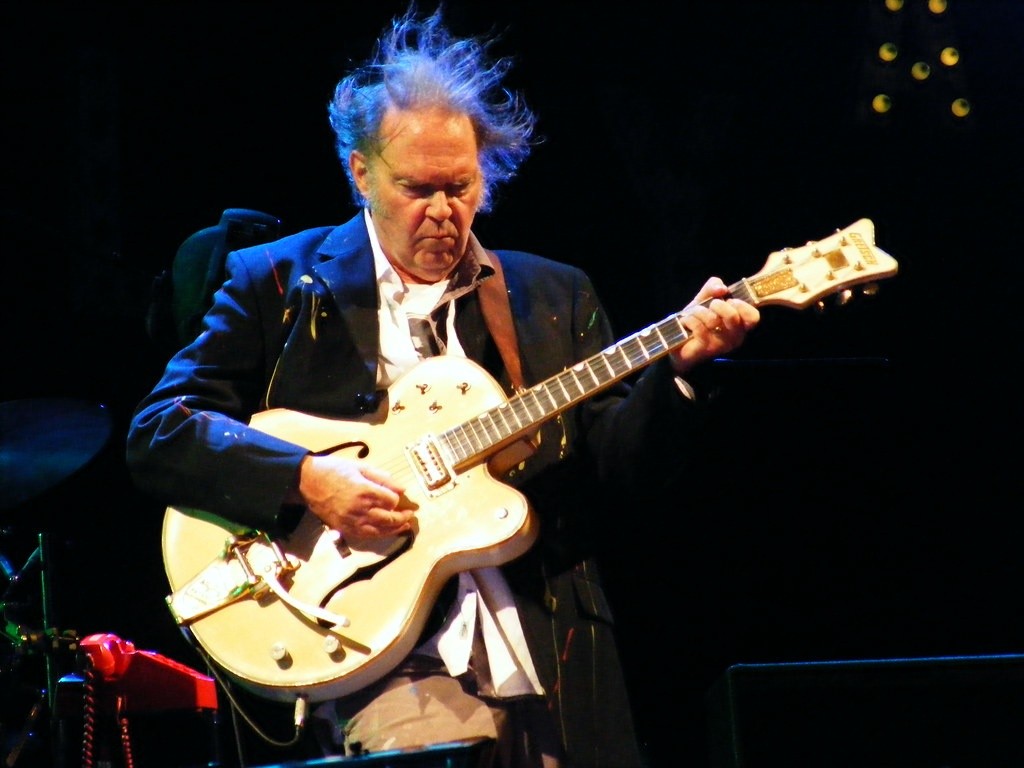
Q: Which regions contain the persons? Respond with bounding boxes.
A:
[126,0,760,768]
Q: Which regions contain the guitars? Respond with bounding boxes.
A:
[161,217,900,704]
